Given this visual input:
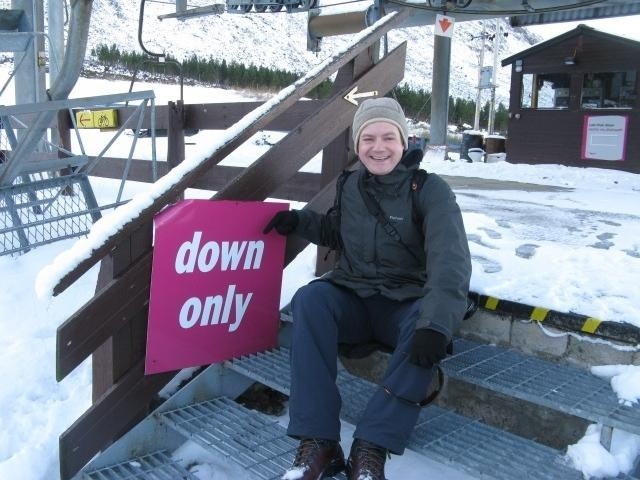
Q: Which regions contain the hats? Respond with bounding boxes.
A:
[352,97,408,156]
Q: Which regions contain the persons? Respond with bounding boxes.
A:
[260,97,472,480]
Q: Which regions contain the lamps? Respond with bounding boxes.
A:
[563,48,577,65]
[515,58,522,73]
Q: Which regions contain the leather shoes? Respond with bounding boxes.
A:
[282,438,345,480]
[345,439,391,480]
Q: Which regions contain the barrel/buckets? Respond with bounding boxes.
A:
[460,129,484,160]
[484,135,507,155]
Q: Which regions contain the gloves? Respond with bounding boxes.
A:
[410,329,448,369]
[263,210,299,235]
